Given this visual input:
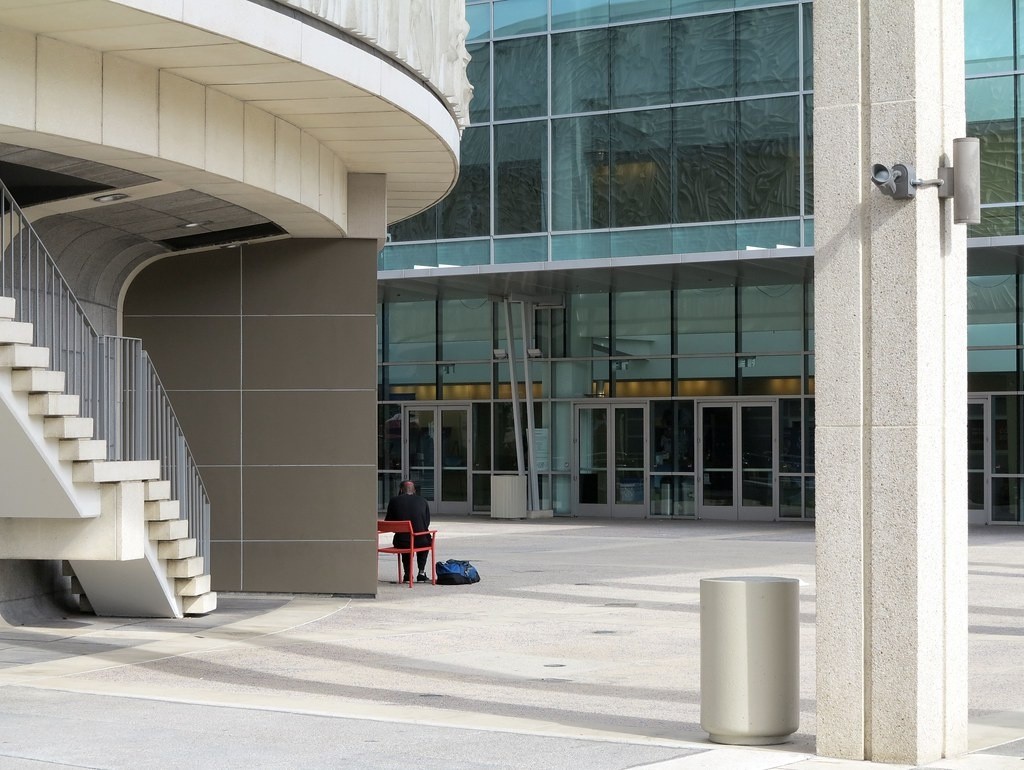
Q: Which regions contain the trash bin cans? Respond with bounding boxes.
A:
[700,577,800,746]
[661,484,674,515]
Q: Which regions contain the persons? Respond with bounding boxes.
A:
[384,480,432,582]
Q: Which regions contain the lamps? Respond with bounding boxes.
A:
[938,136,982,224]
[738,356,756,367]
[528,348,543,358]
[493,349,508,360]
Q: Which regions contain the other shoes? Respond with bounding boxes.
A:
[403,575,410,584]
[416,572,431,583]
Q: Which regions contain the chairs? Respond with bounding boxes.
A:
[377,519,438,588]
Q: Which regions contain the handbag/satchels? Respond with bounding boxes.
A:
[435,559,480,585]
